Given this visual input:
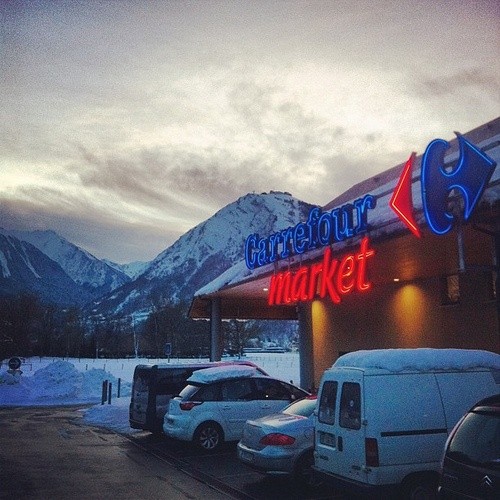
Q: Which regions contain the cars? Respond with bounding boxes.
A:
[167,366,315,453]
[237,396,319,487]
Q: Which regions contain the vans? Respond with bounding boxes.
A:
[435,395,500,500]
[311,349,500,500]
[129,361,273,435]
